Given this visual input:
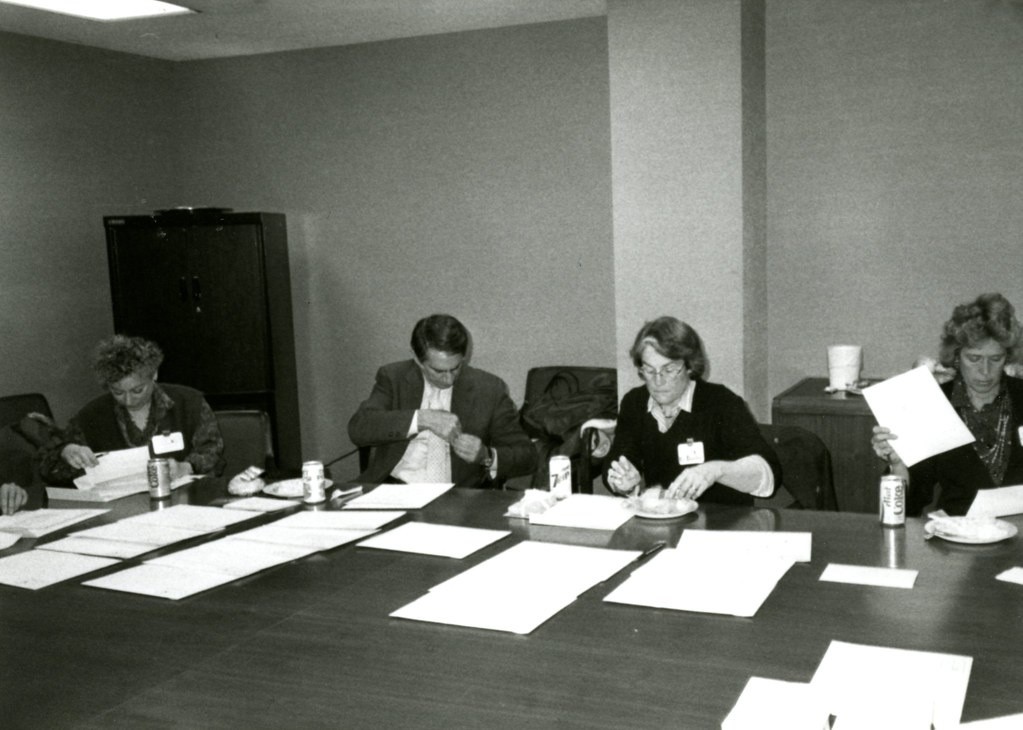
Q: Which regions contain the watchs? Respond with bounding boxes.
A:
[481,447,493,469]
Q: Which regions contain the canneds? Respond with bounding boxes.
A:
[879,475,905,525]
[549,454,572,498]
[302,461,326,504]
[146,457,171,499]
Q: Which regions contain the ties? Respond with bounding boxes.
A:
[429,388,454,482]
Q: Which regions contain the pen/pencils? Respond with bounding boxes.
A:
[633,544,663,564]
[96,452,109,459]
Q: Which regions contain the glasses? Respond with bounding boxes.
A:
[640,363,686,381]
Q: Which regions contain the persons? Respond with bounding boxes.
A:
[0,451,48,514]
[872,292,1023,516]
[350,315,539,489]
[603,318,783,508]
[35,334,224,481]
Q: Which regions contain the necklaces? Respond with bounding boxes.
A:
[962,397,1008,478]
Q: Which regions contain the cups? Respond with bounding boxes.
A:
[828,343,862,389]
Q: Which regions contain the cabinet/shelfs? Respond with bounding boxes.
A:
[105,205,304,481]
[773,375,893,515]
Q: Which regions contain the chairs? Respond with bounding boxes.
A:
[759,425,836,510]
[214,407,277,475]
[522,367,620,497]
[2,394,55,447]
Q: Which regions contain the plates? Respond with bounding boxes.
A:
[629,492,698,518]
[925,516,1018,544]
[263,477,334,497]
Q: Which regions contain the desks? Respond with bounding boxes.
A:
[2,472,1023,722]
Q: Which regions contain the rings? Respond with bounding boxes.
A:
[691,486,698,491]
